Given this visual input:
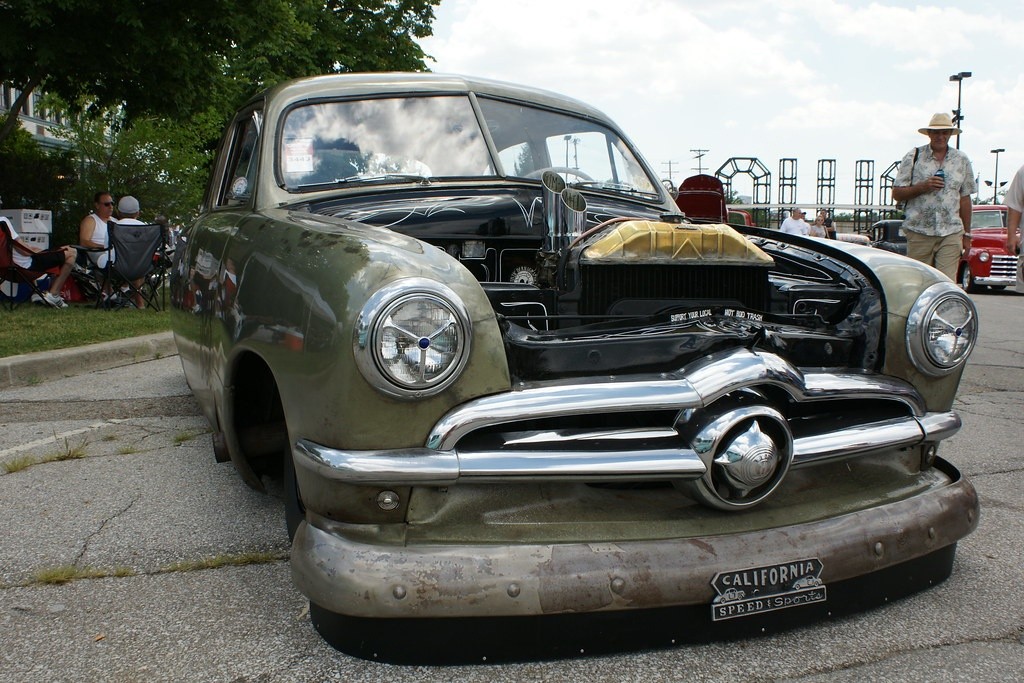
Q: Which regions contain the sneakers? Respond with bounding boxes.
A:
[43,292,68,309]
[31,293,48,306]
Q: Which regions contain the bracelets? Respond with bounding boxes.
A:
[965,232,974,238]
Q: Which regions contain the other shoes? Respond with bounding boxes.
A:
[100,292,118,301]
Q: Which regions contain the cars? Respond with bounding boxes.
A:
[866,219,908,256]
[168,72,978,620]
[956,204,1019,294]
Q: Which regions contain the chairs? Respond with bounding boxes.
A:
[75,221,174,313]
[243,151,364,184]
[0,221,68,312]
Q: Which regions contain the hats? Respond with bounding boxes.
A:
[918,112,963,136]
[118,196,139,213]
[802,212,806,215]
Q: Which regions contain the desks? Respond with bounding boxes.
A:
[50,243,113,302]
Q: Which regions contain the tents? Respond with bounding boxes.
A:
[234,252,338,353]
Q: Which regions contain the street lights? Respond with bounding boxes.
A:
[949,72,972,150]
[563,135,571,183]
[991,148,1006,206]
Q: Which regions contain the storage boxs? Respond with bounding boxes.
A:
[17,234,49,249]
[0,209,53,233]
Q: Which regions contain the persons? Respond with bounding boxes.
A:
[891,113,978,282]
[170,236,238,316]
[1002,163,1024,293]
[79,191,176,310]
[779,208,837,241]
[0,200,76,310]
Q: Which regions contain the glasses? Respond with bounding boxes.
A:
[99,201,116,206]
[817,219,824,221]
